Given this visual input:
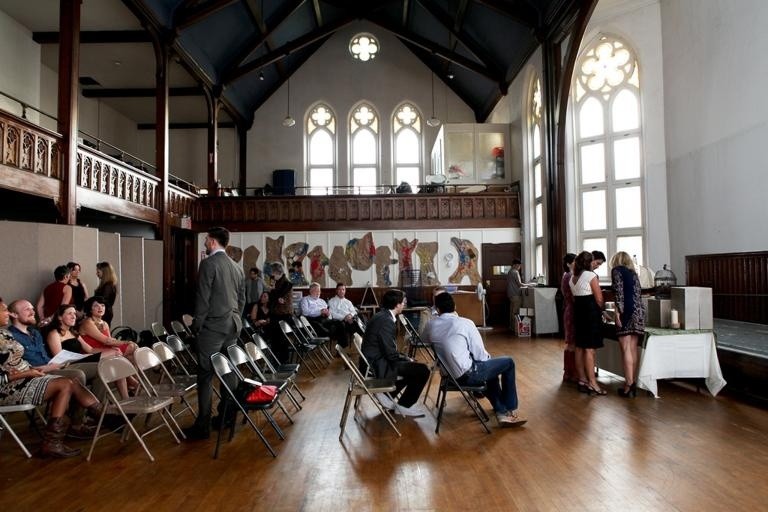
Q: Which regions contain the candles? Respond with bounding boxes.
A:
[671,308,679,324]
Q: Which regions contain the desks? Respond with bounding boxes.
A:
[593,319,715,399]
[520,285,560,338]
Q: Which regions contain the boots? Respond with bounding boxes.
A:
[40,401,104,459]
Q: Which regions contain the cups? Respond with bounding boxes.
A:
[671,310,679,325]
[605,301,615,309]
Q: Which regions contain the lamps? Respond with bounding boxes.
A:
[282,75,295,128]
[427,54,440,128]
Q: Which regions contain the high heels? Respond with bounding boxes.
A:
[617,382,636,398]
[577,379,607,395]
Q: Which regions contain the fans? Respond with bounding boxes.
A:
[477,282,493,330]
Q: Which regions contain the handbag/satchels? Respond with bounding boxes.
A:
[235,377,281,404]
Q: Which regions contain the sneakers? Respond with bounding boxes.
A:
[177,415,233,439]
[495,411,529,427]
[373,392,425,416]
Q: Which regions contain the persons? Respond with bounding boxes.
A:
[1,258,145,458]
[181,225,244,441]
[245,261,365,351]
[589,251,607,272]
[507,256,535,332]
[569,250,609,395]
[421,292,528,427]
[417,288,458,335]
[609,252,647,399]
[358,288,430,417]
[559,253,580,383]
